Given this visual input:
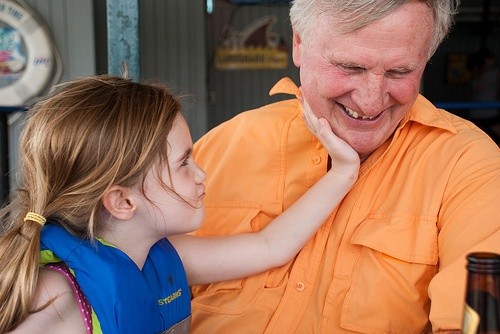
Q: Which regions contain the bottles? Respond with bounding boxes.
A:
[460,250,500,334]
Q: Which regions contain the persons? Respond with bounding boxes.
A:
[0,63,360,334]
[186,0,500,334]
